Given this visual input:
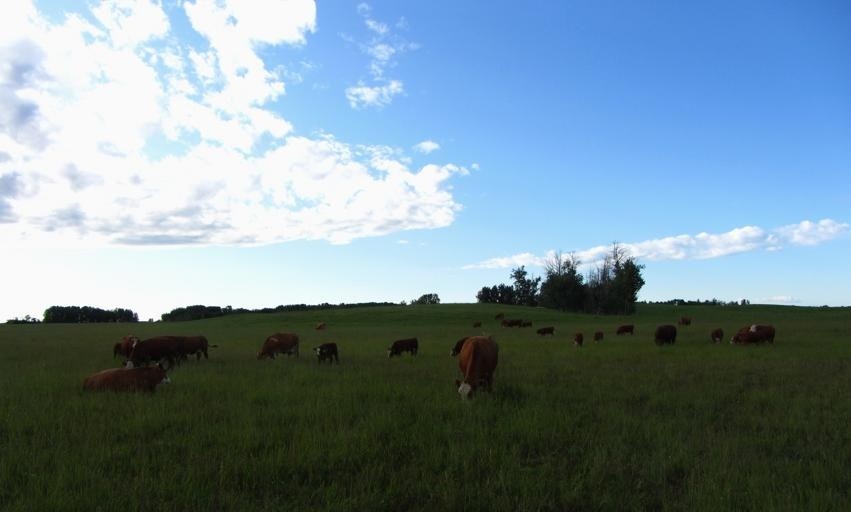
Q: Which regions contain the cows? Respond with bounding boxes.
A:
[385,336,419,360]
[254,331,301,361]
[451,334,500,403]
[537,326,555,337]
[574,332,584,351]
[678,314,693,326]
[594,331,604,340]
[82,333,220,396]
[655,324,677,346]
[493,311,533,328]
[616,324,635,337]
[312,340,341,365]
[711,323,777,347]
